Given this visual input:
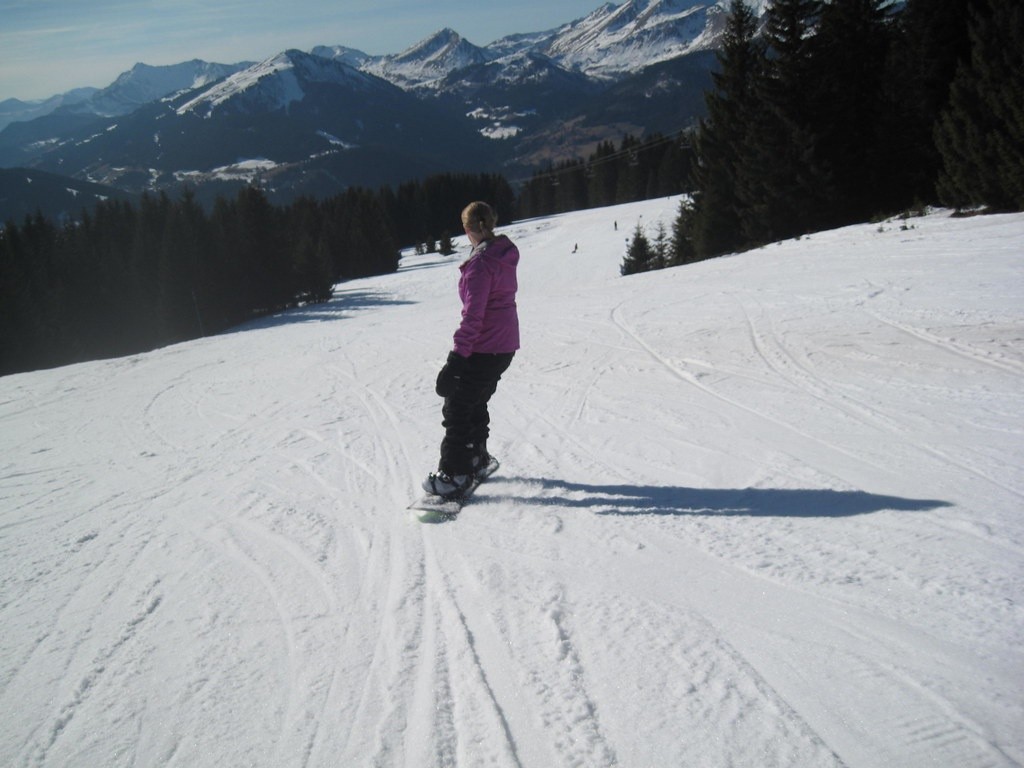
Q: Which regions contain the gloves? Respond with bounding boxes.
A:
[436,349,469,397]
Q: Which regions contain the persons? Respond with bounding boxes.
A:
[421,201,521,496]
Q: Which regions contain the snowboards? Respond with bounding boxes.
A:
[406,451,500,518]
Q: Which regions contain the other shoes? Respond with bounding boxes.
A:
[422,469,472,495]
[464,440,488,469]
